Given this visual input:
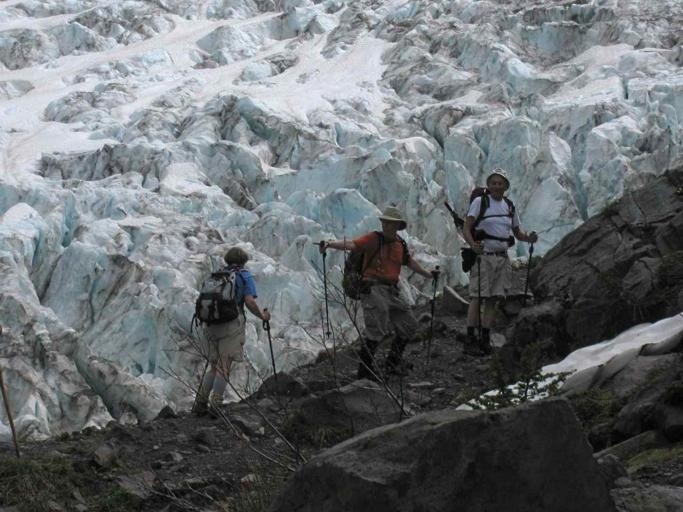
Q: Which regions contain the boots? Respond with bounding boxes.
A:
[385,335,416,374]
[357,336,381,382]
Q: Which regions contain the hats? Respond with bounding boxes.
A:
[377,207,407,230]
[486,168,510,192]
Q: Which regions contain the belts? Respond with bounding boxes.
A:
[487,251,507,255]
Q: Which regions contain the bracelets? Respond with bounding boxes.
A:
[524,231,527,241]
[326,241,330,248]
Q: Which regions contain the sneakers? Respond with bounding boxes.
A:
[463,336,495,357]
[192,400,208,416]
[209,404,232,419]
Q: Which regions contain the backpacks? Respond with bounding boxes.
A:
[342,231,407,300]
[469,187,515,248]
[193,269,247,323]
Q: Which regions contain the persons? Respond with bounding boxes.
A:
[461,168,538,357]
[194,246,271,418]
[310,206,442,383]
